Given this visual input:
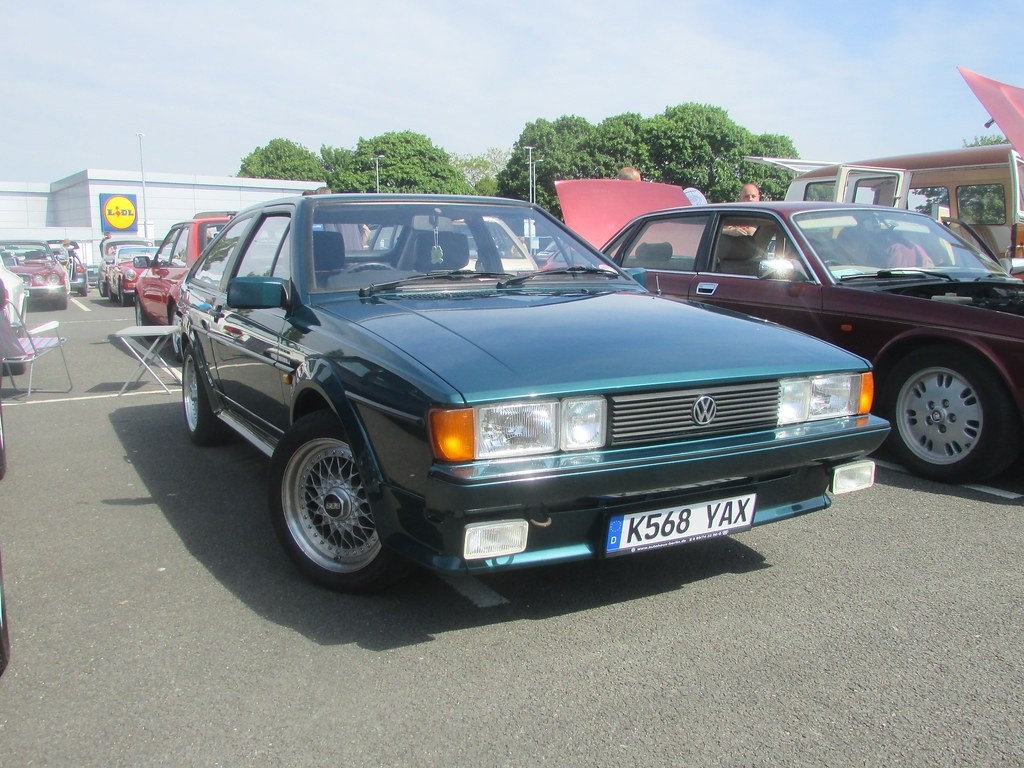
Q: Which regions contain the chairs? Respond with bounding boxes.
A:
[25,250,48,261]
[0,299,73,402]
[1,251,17,266]
[716,231,763,278]
[414,233,469,275]
[312,231,345,286]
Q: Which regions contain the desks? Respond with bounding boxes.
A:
[113,326,182,397]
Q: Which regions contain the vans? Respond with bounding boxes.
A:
[743,144,1024,287]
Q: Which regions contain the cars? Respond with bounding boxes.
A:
[539,177,1023,487]
[97,210,291,363]
[173,191,894,598]
[0,238,87,338]
[447,217,591,274]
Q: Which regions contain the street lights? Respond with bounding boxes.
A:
[524,159,544,205]
[369,154,386,194]
[135,132,147,238]
[524,146,537,202]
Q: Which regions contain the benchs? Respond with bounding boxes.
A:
[623,256,695,271]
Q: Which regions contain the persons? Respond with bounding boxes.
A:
[721,183,761,236]
[876,230,929,268]
[198,295,213,312]
[511,236,528,257]
[302,187,371,250]
[100,232,116,258]
[616,166,641,181]
[63,239,71,247]
